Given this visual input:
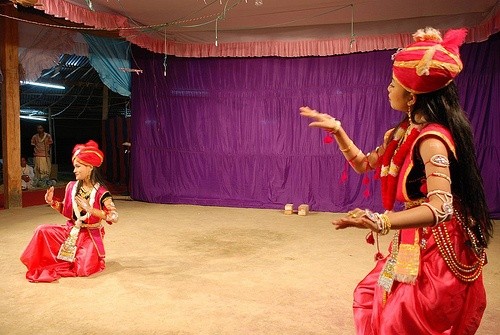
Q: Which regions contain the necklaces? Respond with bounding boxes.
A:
[22,168,26,171]
[38,132,46,142]
[381,119,429,210]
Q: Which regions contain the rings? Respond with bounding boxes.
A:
[347,211,356,218]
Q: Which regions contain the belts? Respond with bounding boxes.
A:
[71,218,101,228]
[405,197,427,210]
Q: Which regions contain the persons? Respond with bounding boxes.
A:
[298,25,495,335]
[19,125,52,191]
[19,138,119,283]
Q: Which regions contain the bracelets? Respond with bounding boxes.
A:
[330,120,362,164]
[93,208,104,219]
[374,212,391,236]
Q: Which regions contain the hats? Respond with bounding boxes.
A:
[391,27,468,94]
[72,140,104,167]
